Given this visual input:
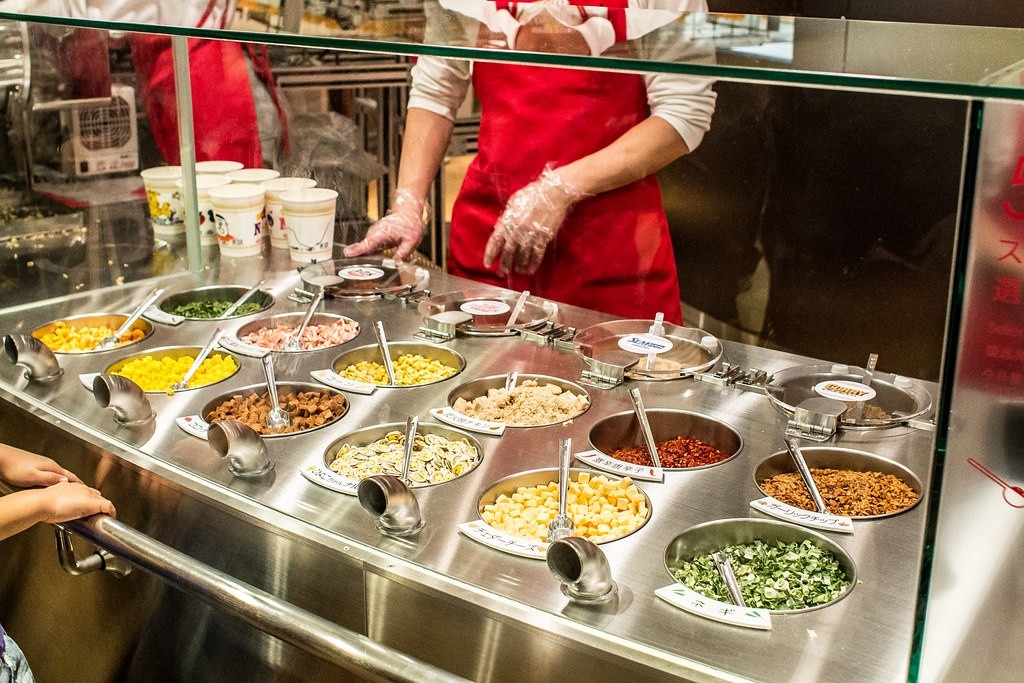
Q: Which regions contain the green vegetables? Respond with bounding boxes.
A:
[675,539,846,611]
[169,300,261,318]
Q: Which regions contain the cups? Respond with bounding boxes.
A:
[209,184,266,256]
[227,169,280,237]
[195,161,244,175]
[140,166,185,235]
[261,178,317,249]
[175,175,232,245]
[279,188,338,262]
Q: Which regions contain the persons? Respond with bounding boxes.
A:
[24,1,292,173]
[955,60,1022,399]
[0,442,116,540]
[344,0,719,326]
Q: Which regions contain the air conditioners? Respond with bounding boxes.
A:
[59,97,138,178]
